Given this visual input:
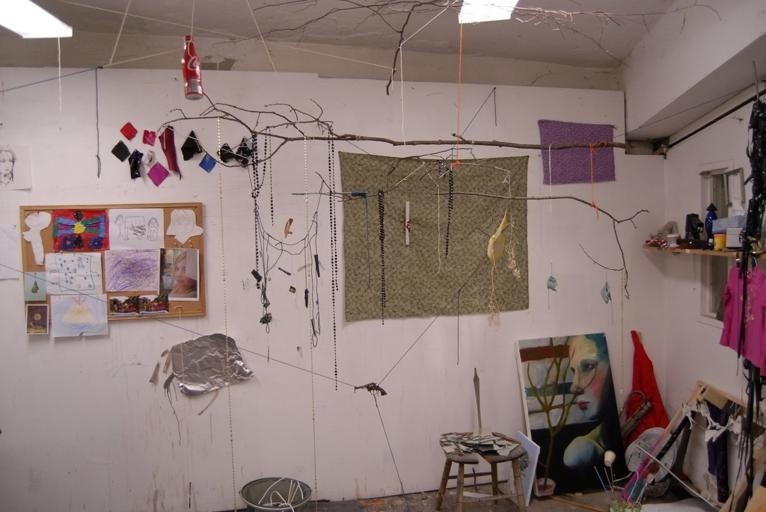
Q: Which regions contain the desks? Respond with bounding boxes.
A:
[436,436,525,512]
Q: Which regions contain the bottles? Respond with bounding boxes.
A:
[180,35,203,99]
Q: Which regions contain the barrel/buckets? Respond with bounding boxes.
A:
[239,477,313,512]
